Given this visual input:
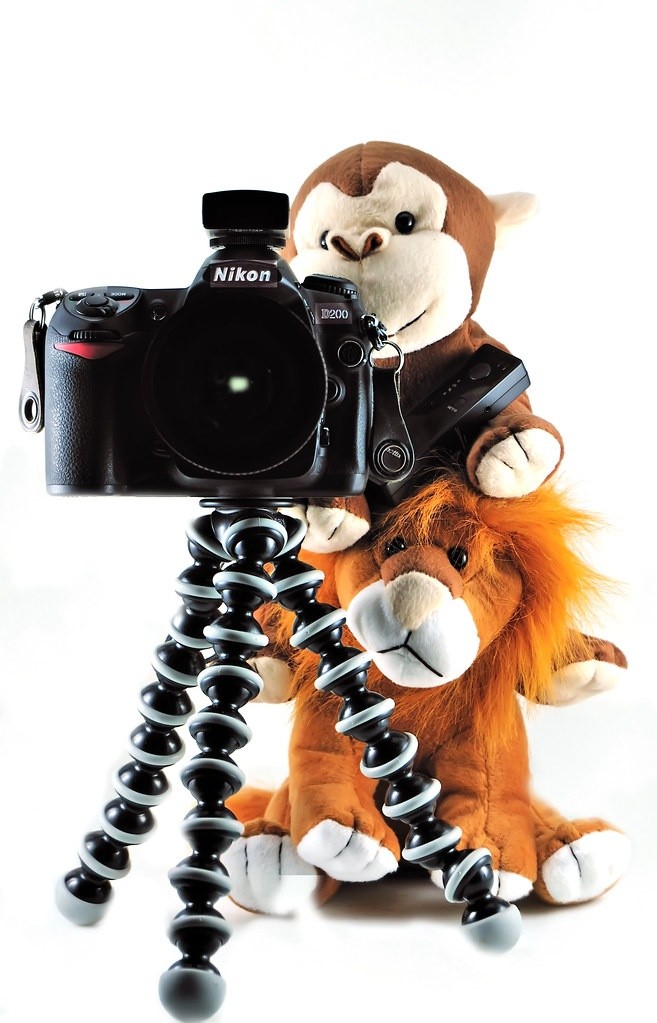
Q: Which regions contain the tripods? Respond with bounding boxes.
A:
[51,502,527,1023]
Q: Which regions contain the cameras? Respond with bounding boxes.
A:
[20,188,417,500]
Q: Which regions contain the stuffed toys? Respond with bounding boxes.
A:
[277,142,564,553]
[218,476,632,914]
